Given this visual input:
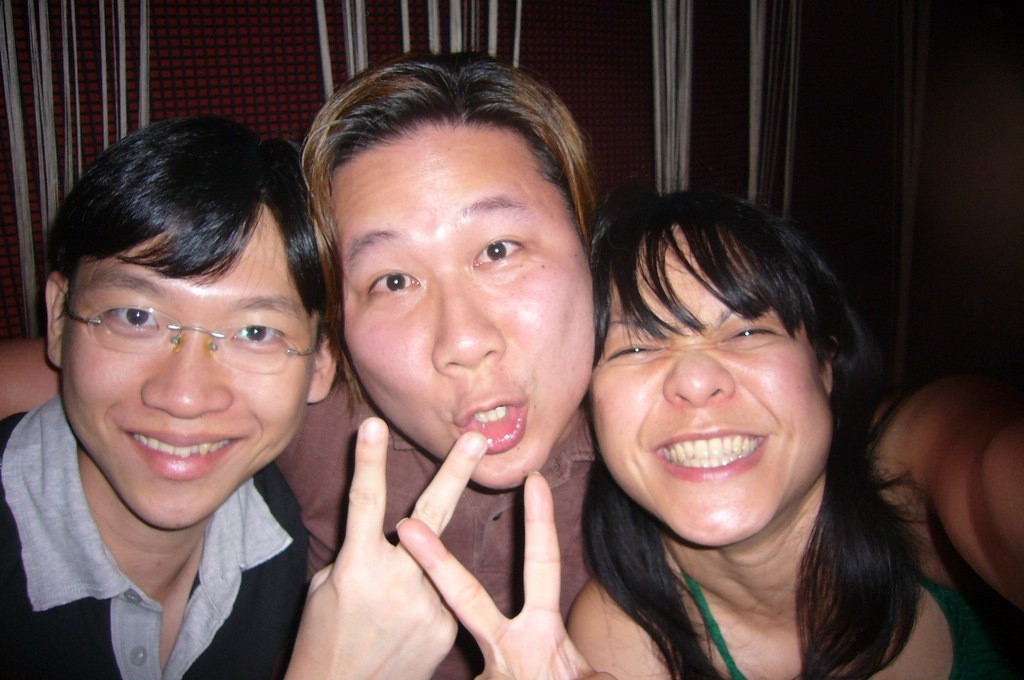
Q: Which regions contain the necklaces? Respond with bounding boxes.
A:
[683,571,745,680]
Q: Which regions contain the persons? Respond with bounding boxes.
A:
[0,52,1024,680]
[395,189,1024,680]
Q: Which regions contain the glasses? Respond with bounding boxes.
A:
[65,290,319,374]
